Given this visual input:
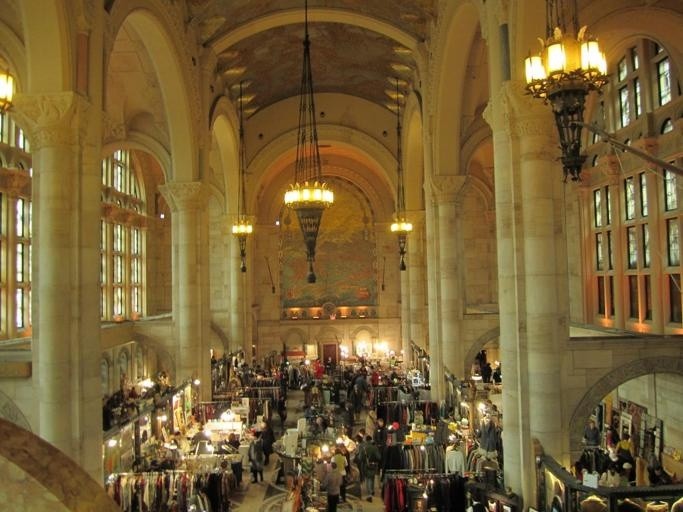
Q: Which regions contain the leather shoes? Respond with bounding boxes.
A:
[367,492,375,502]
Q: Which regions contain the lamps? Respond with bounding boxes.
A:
[522,0,611,187]
[389,76,413,272]
[229,79,256,274]
[283,0,336,285]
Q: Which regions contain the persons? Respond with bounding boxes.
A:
[147,426,211,472]
[108,371,169,417]
[477,351,502,384]
[585,419,666,487]
[229,355,399,512]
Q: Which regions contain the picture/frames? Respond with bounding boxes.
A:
[609,396,662,462]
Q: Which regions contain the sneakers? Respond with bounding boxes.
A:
[264,460,270,466]
[252,478,264,483]
